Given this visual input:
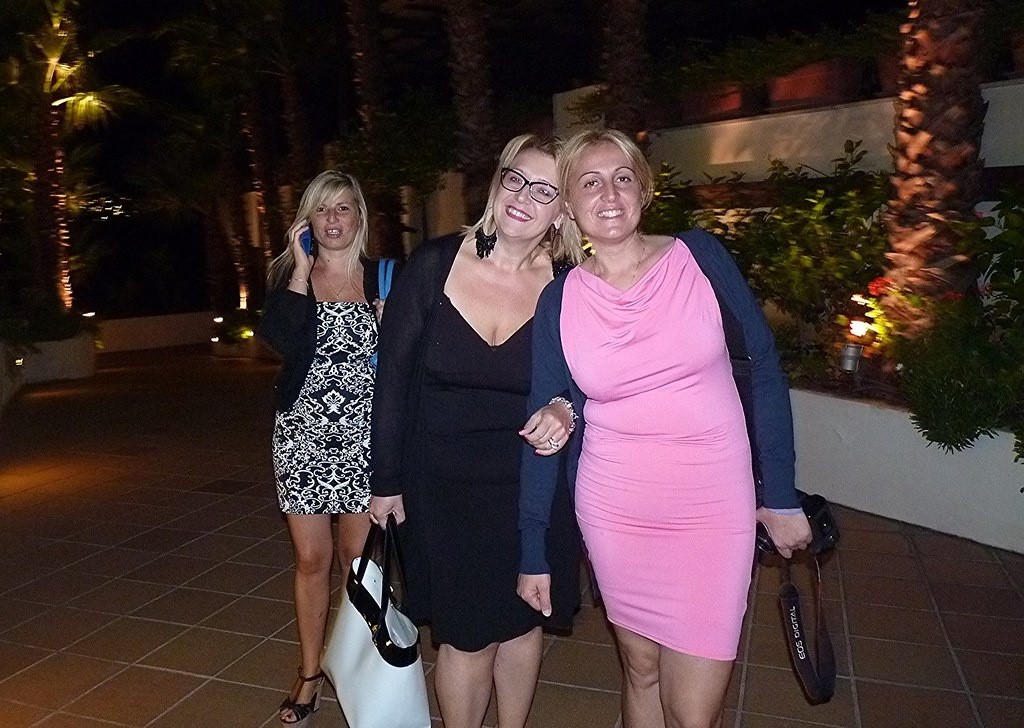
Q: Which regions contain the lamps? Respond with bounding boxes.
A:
[839,343,864,374]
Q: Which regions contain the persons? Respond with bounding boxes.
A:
[249,169,400,728]
[362,131,577,727]
[520,122,815,728]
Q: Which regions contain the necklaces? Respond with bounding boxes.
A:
[592,231,646,287]
[318,260,348,300]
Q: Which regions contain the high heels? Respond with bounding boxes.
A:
[279,666,326,728]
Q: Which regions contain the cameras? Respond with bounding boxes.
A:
[755,488,840,556]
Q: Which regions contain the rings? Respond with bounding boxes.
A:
[369,512,374,514]
[548,437,560,452]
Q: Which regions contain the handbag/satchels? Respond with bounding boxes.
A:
[321,517,431,728]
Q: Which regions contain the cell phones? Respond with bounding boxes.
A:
[301,227,314,257]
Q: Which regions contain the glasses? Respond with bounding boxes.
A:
[500,167,559,205]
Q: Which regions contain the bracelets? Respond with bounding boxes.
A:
[548,396,580,435]
[762,506,803,514]
[288,278,309,291]
